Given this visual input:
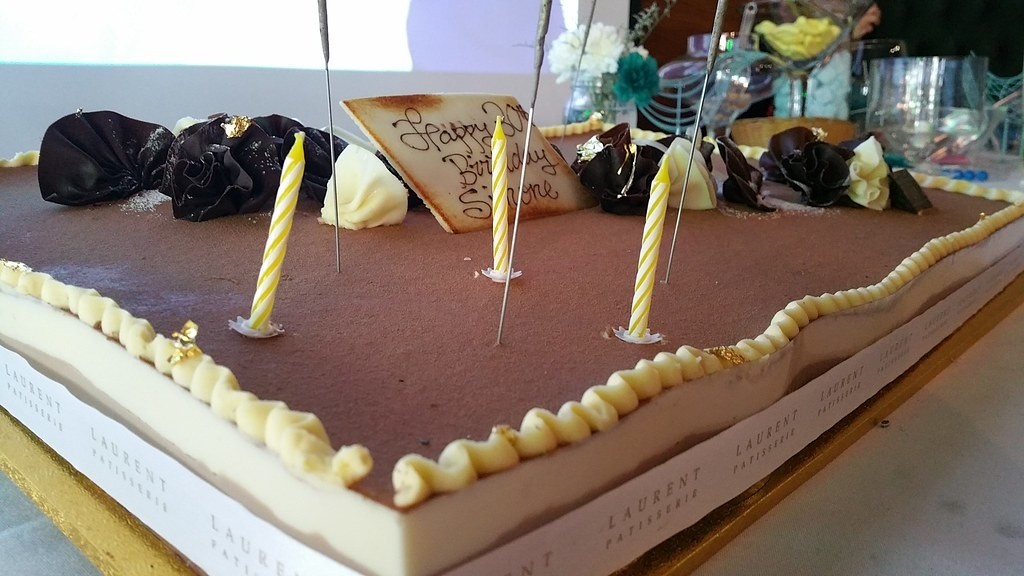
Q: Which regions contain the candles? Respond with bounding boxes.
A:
[488,114,513,277]
[626,154,672,339]
[247,130,307,329]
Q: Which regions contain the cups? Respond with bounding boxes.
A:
[860,57,947,156]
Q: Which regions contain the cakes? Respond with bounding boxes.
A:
[0,93,1023,576]
